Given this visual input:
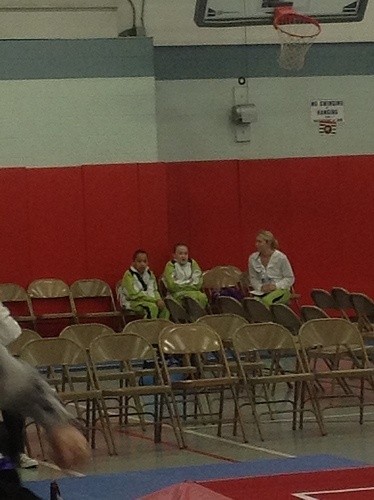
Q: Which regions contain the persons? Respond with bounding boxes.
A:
[248,228,294,308]
[0,303,98,500]
[116,248,170,322]
[159,244,207,312]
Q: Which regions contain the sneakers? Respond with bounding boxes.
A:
[15,453,38,469]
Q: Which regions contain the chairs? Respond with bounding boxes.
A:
[0,265,374,461]
